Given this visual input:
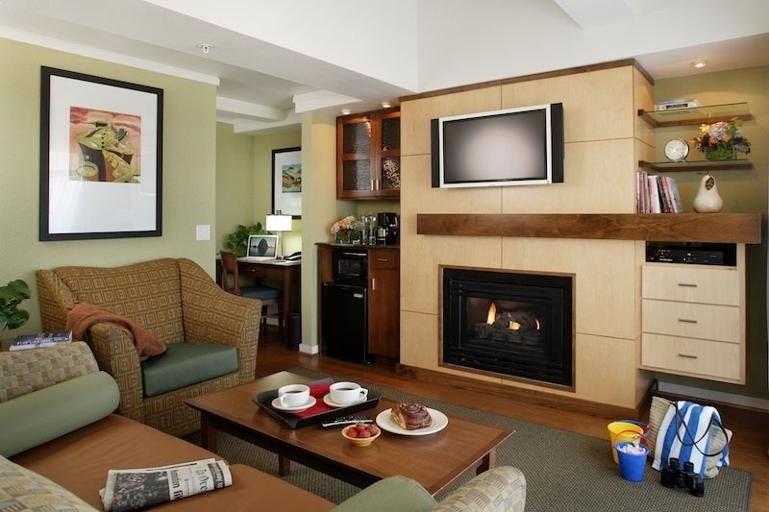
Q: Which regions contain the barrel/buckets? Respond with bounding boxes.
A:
[612,430,652,481]
[607,420,649,465]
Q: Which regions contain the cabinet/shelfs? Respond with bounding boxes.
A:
[637,98,755,174]
[337,105,401,200]
[640,241,750,385]
[314,242,400,374]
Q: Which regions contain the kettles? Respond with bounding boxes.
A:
[378,212,398,246]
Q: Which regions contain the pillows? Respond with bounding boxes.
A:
[0,370,121,459]
[326,475,437,512]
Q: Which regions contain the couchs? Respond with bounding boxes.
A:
[0,341,527,512]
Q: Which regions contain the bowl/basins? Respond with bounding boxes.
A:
[342,424,382,448]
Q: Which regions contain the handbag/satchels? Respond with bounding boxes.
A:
[642,396,733,479]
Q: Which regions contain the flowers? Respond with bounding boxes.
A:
[688,114,751,153]
[331,215,354,233]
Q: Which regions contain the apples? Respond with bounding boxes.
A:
[347,423,379,439]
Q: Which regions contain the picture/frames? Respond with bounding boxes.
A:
[39,65,162,241]
[271,146,302,219]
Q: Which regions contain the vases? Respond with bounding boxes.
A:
[337,229,348,243]
[705,148,737,160]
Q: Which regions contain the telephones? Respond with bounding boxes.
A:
[286,251,301,260]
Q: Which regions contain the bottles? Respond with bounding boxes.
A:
[352,210,386,245]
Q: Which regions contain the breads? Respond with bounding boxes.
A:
[391,402,432,430]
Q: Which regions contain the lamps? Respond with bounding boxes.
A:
[265,210,292,260]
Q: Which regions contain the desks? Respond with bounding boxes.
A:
[216,257,301,329]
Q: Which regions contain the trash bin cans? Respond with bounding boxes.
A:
[286,311,301,351]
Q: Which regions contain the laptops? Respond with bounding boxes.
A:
[239,235,279,261]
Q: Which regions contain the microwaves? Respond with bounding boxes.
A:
[331,247,369,285]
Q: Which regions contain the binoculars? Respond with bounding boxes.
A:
[660,458,704,497]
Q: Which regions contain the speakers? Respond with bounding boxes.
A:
[321,282,368,365]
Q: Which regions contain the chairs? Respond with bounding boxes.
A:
[220,250,282,333]
[34,258,261,437]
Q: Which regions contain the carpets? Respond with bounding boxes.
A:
[178,365,751,512]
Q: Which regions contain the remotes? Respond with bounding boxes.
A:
[319,415,373,427]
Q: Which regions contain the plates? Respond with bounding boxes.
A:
[322,392,368,409]
[377,405,450,435]
[269,394,317,414]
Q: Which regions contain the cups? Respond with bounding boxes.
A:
[278,383,310,405]
[329,381,368,403]
[74,162,100,182]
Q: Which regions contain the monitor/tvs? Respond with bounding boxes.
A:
[431,104,564,189]
